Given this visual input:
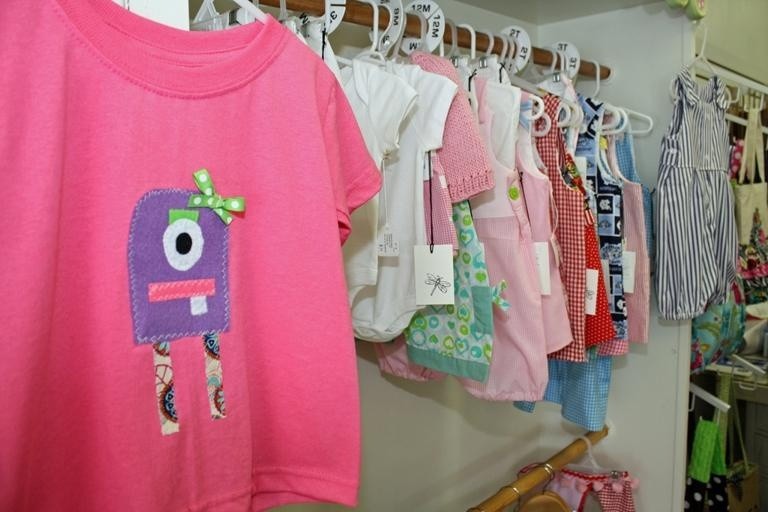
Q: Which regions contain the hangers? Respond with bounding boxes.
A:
[562,434,619,475]
[466,461,572,511]
[668,17,731,109]
